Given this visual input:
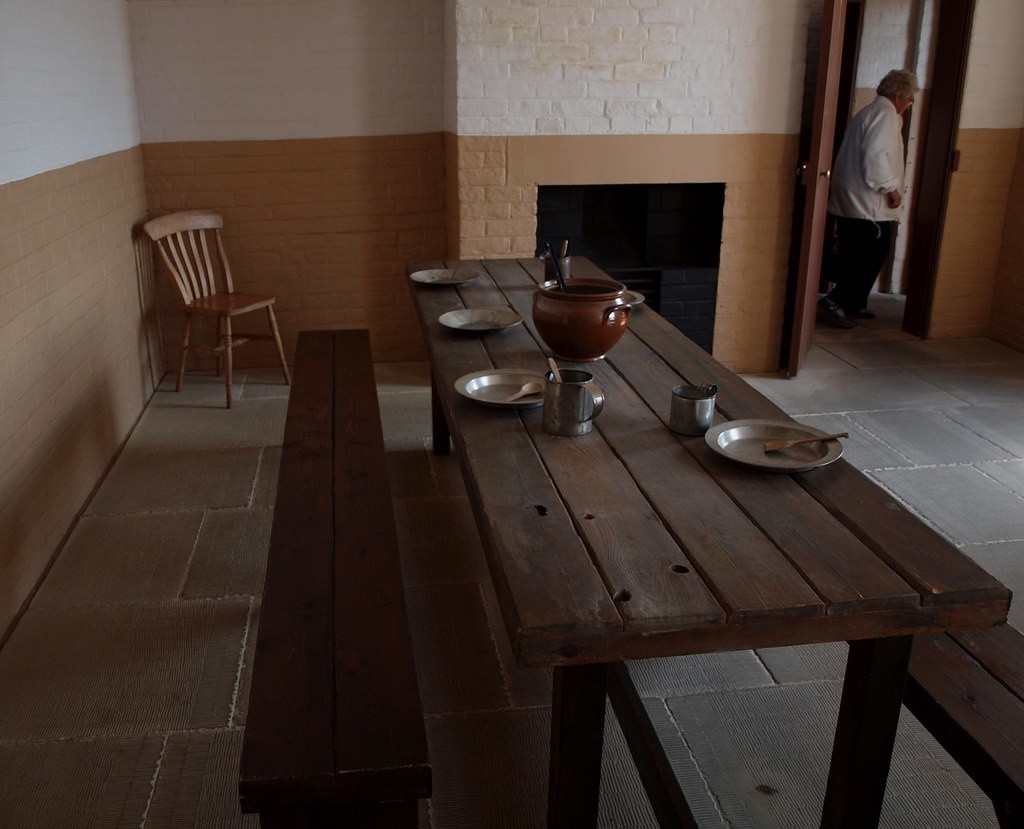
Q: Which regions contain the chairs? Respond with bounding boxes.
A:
[144,208,292,410]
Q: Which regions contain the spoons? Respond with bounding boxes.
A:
[504,382,542,401]
[761,432,849,452]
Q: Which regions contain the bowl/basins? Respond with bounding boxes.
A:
[532,277,633,362]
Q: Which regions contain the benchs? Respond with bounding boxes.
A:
[237,329,432,829]
[849,555,1024,829]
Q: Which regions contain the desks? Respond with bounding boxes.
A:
[403,255,1014,829]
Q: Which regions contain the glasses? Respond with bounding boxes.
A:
[906,96,915,106]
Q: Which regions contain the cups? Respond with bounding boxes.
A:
[544,253,571,281]
[542,368,605,437]
[668,381,719,438]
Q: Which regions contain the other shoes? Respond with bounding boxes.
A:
[852,307,878,320]
[816,296,856,329]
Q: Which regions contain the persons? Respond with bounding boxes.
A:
[817,70,920,329]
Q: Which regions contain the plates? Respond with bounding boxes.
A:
[438,307,524,331]
[454,366,547,406]
[627,291,645,304]
[409,268,481,286]
[703,418,844,473]
